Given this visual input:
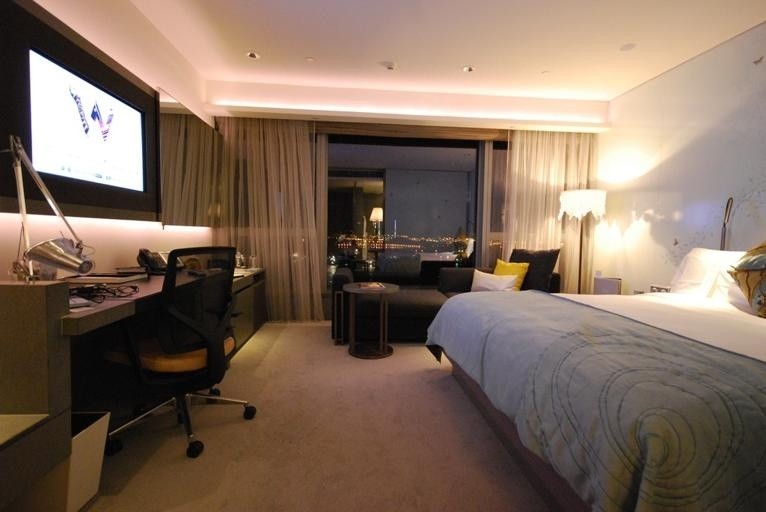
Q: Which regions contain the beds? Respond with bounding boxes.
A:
[424,248,766,512]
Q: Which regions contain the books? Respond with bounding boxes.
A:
[61,271,149,285]
[358,281,385,290]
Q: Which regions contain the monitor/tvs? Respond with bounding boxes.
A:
[18,44,150,199]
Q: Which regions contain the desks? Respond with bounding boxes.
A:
[343,280,400,359]
[0,266,265,512]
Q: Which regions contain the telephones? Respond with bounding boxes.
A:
[137,248,185,275]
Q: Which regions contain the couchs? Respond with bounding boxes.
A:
[332,262,560,344]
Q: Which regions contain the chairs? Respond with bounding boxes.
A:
[107,245,258,459]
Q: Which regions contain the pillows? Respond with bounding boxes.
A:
[471,267,518,291]
[493,255,532,291]
[511,247,561,290]
[727,242,766,318]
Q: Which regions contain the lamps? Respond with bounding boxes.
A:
[558,188,605,294]
[370,207,384,222]
